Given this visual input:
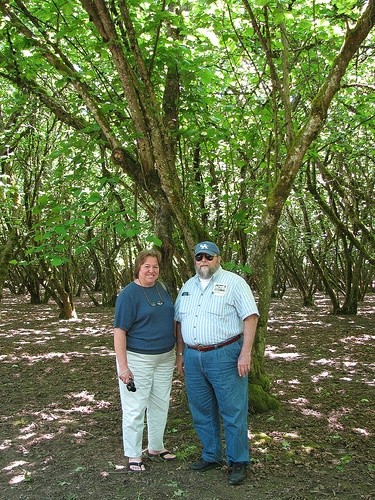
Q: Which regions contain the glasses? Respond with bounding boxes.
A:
[195,254,218,261]
[149,300,164,307]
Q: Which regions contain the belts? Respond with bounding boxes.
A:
[187,334,241,351]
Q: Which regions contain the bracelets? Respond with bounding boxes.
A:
[175,352,183,355]
[117,369,131,378]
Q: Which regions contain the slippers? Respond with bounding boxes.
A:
[129,458,146,471]
[149,450,176,460]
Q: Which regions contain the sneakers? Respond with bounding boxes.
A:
[229,462,246,484]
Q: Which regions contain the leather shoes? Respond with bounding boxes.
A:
[188,456,223,472]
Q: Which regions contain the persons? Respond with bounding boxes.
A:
[113,247,177,471]
[173,241,260,485]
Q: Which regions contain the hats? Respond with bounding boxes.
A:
[194,241,220,257]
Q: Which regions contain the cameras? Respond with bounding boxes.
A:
[127,378,136,392]
